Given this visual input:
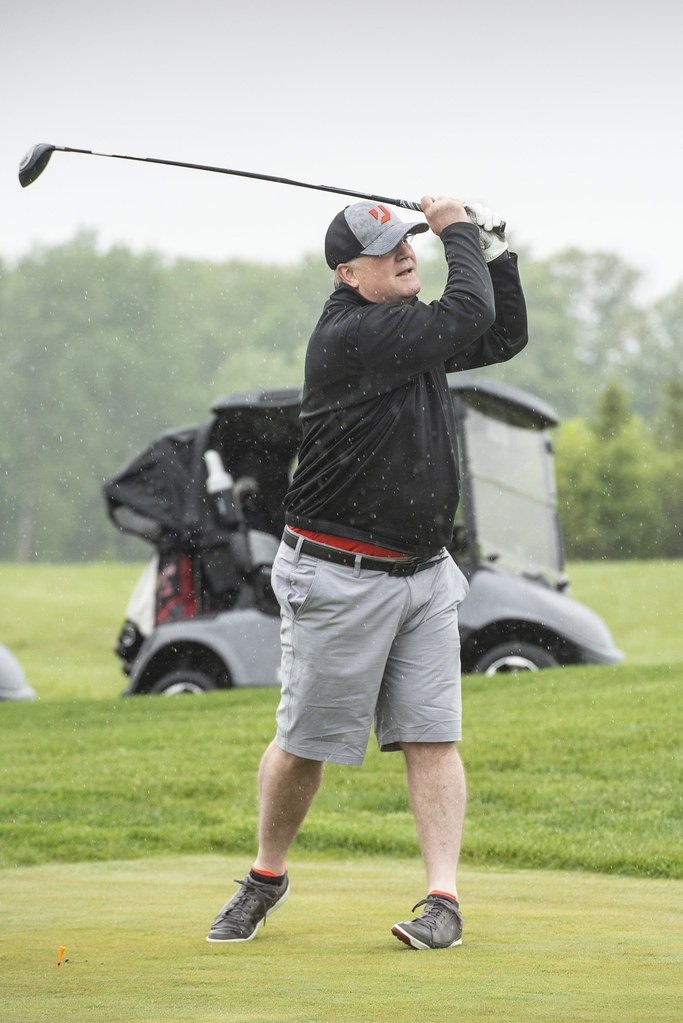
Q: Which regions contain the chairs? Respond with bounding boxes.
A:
[232,422,304,573]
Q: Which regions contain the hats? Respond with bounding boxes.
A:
[325,203,429,271]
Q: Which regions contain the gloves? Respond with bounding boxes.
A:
[464,200,509,263]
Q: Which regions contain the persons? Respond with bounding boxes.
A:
[206,196,527,949]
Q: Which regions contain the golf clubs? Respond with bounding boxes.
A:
[18,143,506,233]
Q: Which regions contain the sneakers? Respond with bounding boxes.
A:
[390,892,464,952]
[207,874,290,943]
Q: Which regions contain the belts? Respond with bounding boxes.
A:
[282,527,448,577]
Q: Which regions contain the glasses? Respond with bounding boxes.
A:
[355,233,414,259]
